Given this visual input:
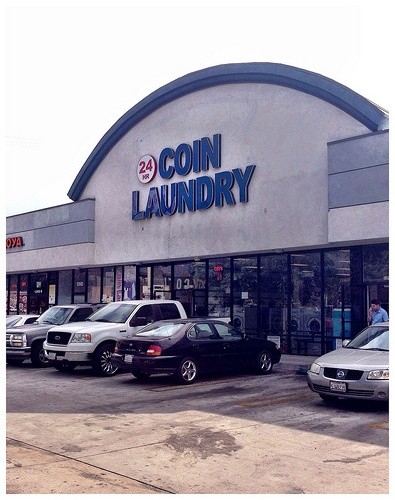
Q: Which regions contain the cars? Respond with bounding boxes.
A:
[306,322,389,405]
[5,314,41,330]
[110,317,282,385]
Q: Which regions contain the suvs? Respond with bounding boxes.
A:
[6,302,106,369]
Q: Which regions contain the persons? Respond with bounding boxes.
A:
[367,298,388,325]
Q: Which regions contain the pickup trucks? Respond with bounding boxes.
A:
[42,299,233,377]
